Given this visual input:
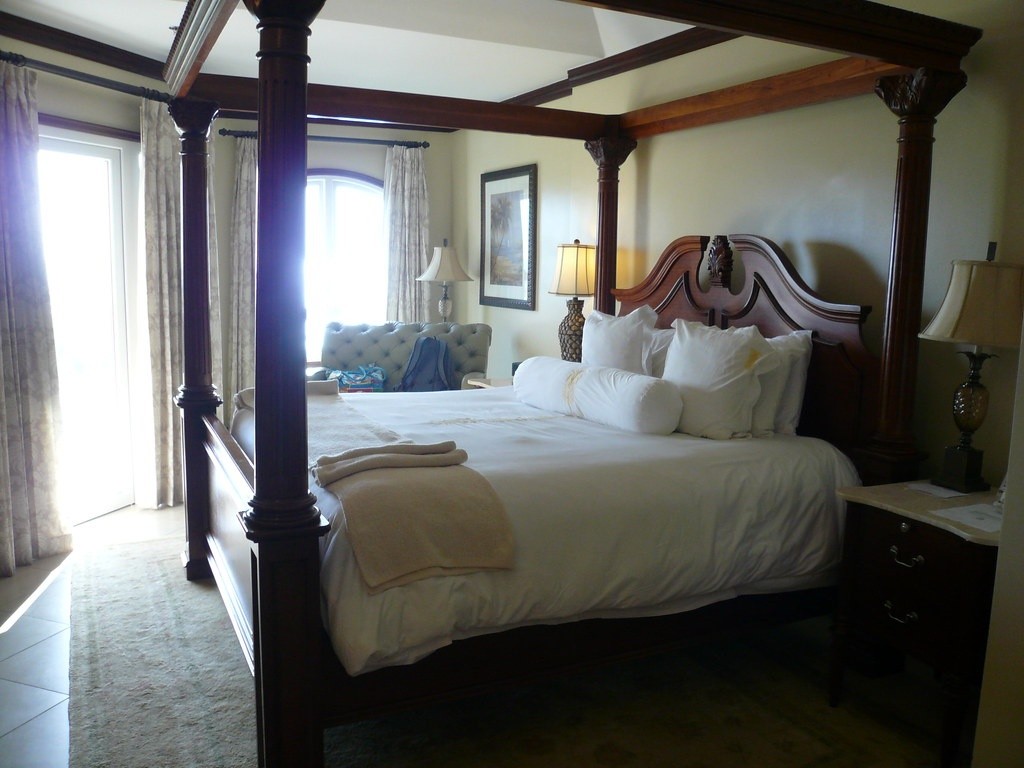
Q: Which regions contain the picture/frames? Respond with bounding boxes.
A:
[479,163,536,312]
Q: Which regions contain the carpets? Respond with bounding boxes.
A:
[67,535,953,768]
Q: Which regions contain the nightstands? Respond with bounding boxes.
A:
[835,480,1008,758]
[467,377,515,389]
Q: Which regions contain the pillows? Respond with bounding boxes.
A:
[513,355,584,416]
[581,304,658,376]
[569,365,683,435]
[651,318,812,441]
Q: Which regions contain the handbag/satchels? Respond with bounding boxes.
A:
[329,365,385,393]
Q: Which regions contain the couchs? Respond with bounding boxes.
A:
[309,322,492,391]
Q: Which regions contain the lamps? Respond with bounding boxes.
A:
[548,239,597,361]
[918,240,1024,494]
[416,239,474,322]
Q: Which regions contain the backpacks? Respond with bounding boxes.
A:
[401,336,452,391]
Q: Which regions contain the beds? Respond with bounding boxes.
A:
[231,233,870,727]
